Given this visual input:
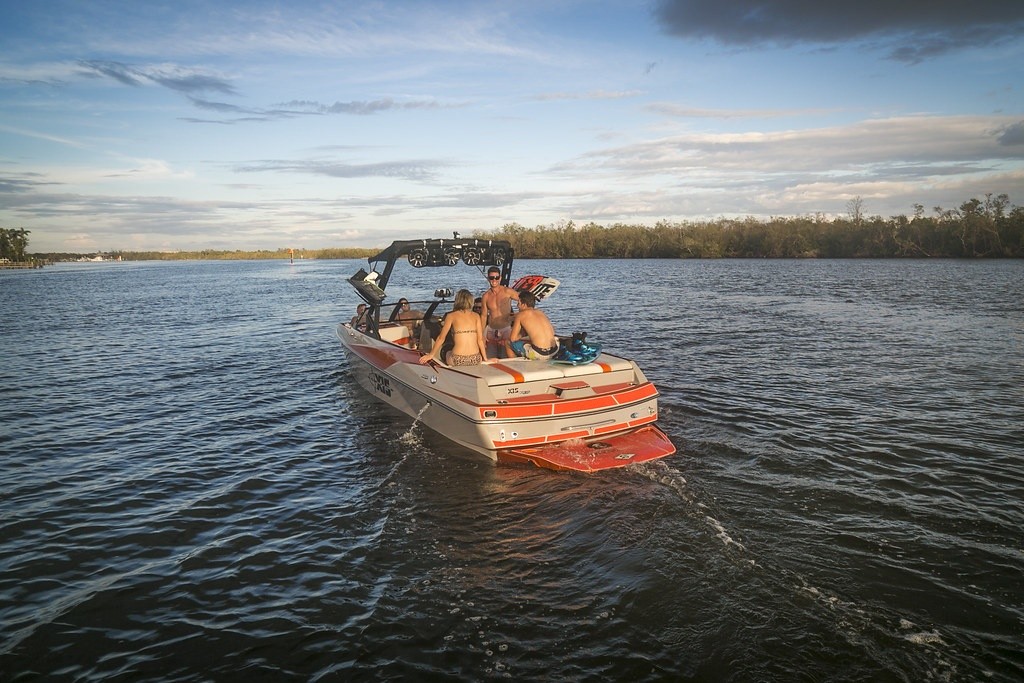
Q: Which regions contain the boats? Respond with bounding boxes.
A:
[335,232,681,478]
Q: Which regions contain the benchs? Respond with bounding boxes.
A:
[451,352,635,399]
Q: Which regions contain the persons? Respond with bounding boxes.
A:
[394,298,425,350]
[506,290,561,363]
[480,266,521,360]
[473,297,491,326]
[351,304,371,332]
[419,289,500,367]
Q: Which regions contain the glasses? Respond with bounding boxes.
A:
[488,276,501,280]
